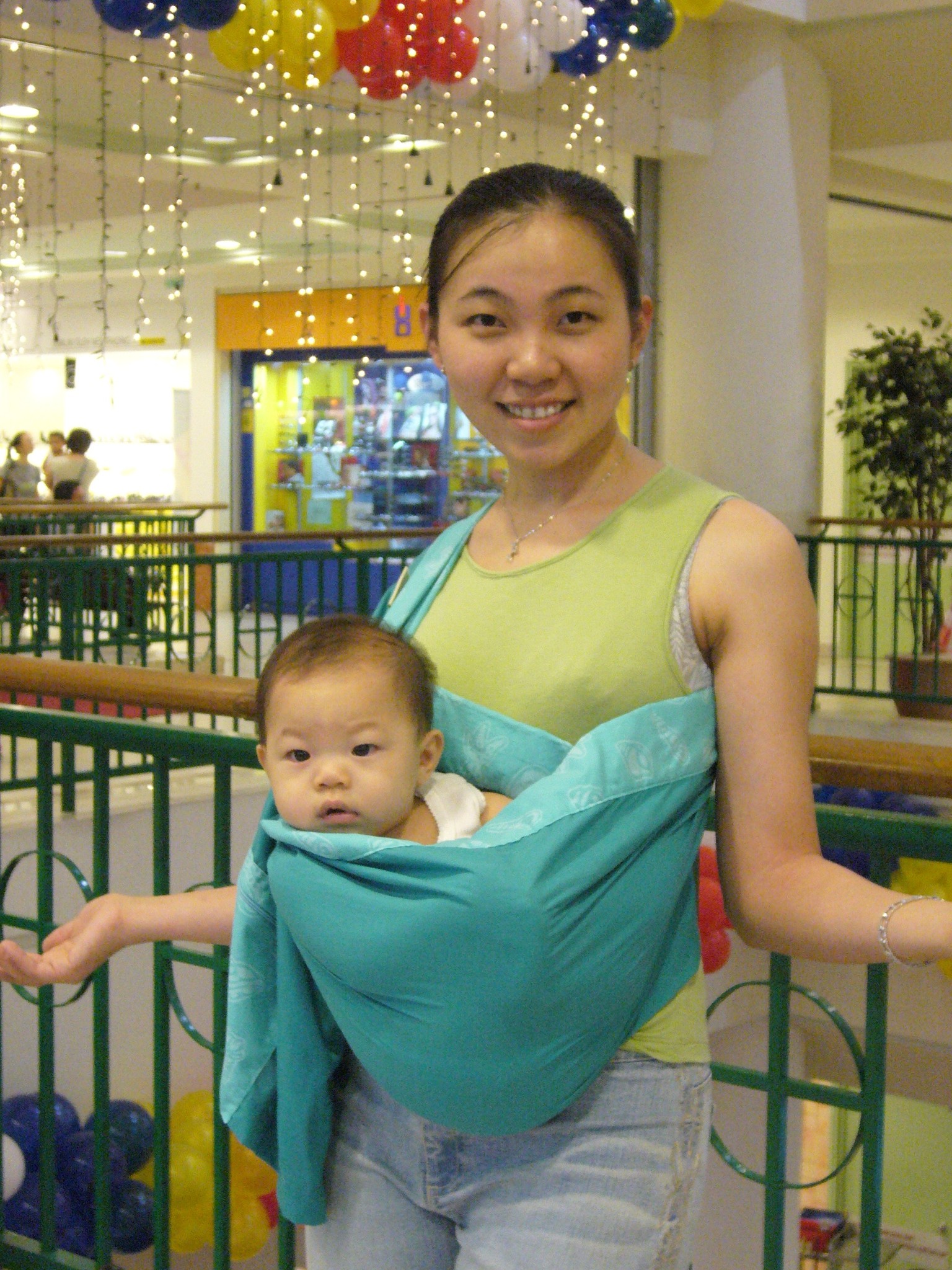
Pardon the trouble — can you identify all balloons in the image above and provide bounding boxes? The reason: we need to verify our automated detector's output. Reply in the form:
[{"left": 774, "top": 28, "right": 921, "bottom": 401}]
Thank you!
[{"left": 86, "top": 0, "right": 724, "bottom": 99}]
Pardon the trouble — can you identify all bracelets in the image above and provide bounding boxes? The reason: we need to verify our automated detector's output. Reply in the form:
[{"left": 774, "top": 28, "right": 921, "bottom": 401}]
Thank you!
[{"left": 875, "top": 892, "right": 940, "bottom": 977}]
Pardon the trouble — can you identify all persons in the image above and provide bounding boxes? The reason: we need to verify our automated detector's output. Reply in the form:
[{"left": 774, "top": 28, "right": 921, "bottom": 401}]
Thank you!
[
  {"left": 0, "top": 427, "right": 99, "bottom": 555},
  {"left": 0, "top": 155, "right": 952, "bottom": 1270},
  {"left": 252, "top": 615, "right": 522, "bottom": 857}
]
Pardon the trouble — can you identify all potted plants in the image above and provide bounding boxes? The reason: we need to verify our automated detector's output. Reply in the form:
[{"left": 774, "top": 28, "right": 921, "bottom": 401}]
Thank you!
[{"left": 828, "top": 306, "right": 952, "bottom": 720}]
